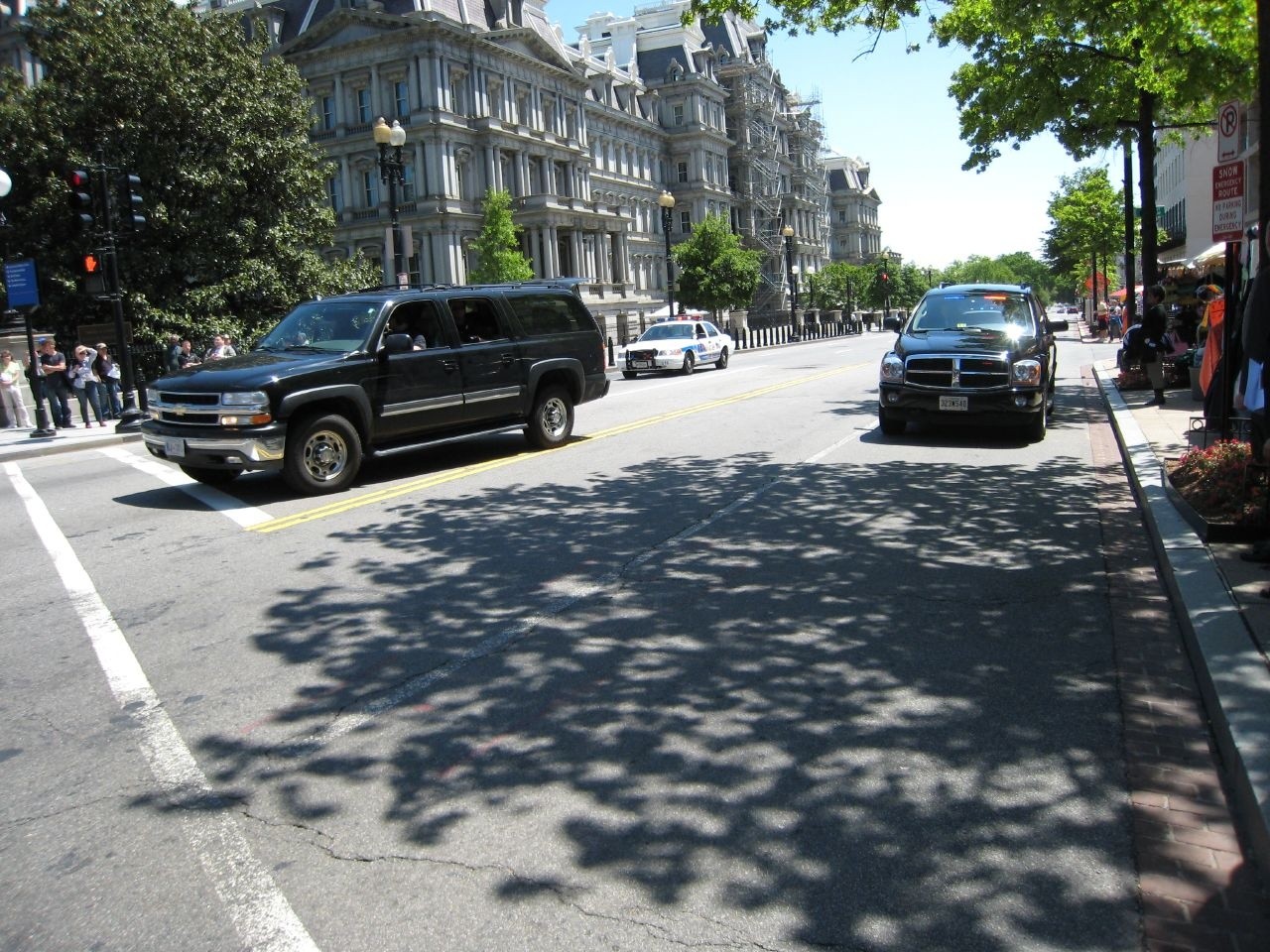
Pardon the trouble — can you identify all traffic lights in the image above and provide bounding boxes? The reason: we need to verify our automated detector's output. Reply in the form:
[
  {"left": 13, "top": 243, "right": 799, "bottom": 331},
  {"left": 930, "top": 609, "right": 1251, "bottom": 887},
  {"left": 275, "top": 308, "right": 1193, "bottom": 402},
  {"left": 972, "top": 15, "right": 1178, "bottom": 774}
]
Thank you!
[
  {"left": 81, "top": 252, "right": 107, "bottom": 292},
  {"left": 118, "top": 173, "right": 151, "bottom": 234},
  {"left": 68, "top": 169, "right": 99, "bottom": 234}
]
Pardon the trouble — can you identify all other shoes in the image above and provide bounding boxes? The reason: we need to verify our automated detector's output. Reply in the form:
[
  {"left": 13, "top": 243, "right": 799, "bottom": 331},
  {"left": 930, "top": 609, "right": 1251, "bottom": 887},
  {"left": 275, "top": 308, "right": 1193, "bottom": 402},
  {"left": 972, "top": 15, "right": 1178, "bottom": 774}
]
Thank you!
[
  {"left": 1145, "top": 399, "right": 1165, "bottom": 405},
  {"left": 102, "top": 413, "right": 111, "bottom": 421},
  {"left": 114, "top": 414, "right": 123, "bottom": 419},
  {"left": 86, "top": 422, "right": 91, "bottom": 428},
  {"left": 100, "top": 420, "right": 106, "bottom": 426},
  {"left": 56, "top": 423, "right": 63, "bottom": 429},
  {"left": 63, "top": 423, "right": 76, "bottom": 427}
]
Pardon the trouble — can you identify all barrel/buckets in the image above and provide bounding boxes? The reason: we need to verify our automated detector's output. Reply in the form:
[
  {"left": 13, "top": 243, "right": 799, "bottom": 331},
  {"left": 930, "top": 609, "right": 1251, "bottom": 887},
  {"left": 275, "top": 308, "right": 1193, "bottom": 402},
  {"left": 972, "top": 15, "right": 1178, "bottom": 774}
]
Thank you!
[{"left": 1188, "top": 365, "right": 1203, "bottom": 401}]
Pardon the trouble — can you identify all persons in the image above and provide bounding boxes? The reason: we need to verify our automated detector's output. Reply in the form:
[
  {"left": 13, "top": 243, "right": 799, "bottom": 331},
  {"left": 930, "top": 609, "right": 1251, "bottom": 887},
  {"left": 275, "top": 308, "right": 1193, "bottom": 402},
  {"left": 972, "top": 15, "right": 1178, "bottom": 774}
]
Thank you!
[
  {"left": 177, "top": 340, "right": 202, "bottom": 369},
  {"left": 1180, "top": 302, "right": 1205, "bottom": 344},
  {"left": 163, "top": 335, "right": 182, "bottom": 374},
  {"left": 67, "top": 345, "right": 107, "bottom": 429},
  {"left": 0, "top": 350, "right": 34, "bottom": 429},
  {"left": 1106, "top": 300, "right": 1128, "bottom": 343},
  {"left": 23, "top": 338, "right": 78, "bottom": 430},
  {"left": 93, "top": 343, "right": 123, "bottom": 421},
  {"left": 204, "top": 335, "right": 238, "bottom": 363},
  {"left": 1141, "top": 285, "right": 1169, "bottom": 404}
]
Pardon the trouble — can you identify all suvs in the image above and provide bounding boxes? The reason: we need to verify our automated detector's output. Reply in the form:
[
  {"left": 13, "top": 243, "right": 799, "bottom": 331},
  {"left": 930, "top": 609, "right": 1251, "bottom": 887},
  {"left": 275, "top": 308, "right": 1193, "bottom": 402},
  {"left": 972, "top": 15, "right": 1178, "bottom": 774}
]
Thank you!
[
  {"left": 143, "top": 279, "right": 615, "bottom": 497},
  {"left": 879, "top": 281, "right": 1069, "bottom": 445}
]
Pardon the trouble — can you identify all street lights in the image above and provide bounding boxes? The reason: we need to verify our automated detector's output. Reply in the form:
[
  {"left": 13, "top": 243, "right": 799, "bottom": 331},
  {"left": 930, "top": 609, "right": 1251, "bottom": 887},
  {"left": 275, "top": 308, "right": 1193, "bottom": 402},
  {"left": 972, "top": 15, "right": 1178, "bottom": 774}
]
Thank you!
[
  {"left": 782, "top": 223, "right": 802, "bottom": 342},
  {"left": 658, "top": 190, "right": 676, "bottom": 318},
  {"left": 880, "top": 250, "right": 896, "bottom": 320},
  {"left": 372, "top": 116, "right": 409, "bottom": 287}
]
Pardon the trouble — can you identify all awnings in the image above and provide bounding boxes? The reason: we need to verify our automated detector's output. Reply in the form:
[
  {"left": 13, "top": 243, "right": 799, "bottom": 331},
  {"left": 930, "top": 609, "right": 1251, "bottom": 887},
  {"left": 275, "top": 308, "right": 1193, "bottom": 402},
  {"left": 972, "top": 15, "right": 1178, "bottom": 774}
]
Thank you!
[
  {"left": 1110, "top": 286, "right": 1143, "bottom": 304},
  {"left": 1157, "top": 240, "right": 1225, "bottom": 280}
]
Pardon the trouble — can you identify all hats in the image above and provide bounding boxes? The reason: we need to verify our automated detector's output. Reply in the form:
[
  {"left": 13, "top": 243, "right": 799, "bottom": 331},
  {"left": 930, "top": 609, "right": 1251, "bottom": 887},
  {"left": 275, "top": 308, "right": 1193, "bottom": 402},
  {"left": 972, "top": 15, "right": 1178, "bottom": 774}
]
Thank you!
[
  {"left": 94, "top": 342, "right": 109, "bottom": 349},
  {"left": 38, "top": 336, "right": 48, "bottom": 345},
  {"left": 75, "top": 345, "right": 89, "bottom": 357},
  {"left": 1147, "top": 285, "right": 1167, "bottom": 296}
]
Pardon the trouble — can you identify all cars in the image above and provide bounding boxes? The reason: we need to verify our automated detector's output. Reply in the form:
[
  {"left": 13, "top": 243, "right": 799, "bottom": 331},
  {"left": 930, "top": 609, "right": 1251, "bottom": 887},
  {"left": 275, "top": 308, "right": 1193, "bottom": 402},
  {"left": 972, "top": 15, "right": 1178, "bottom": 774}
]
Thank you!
[
  {"left": 616, "top": 314, "right": 737, "bottom": 379},
  {"left": 1055, "top": 305, "right": 1079, "bottom": 315}
]
